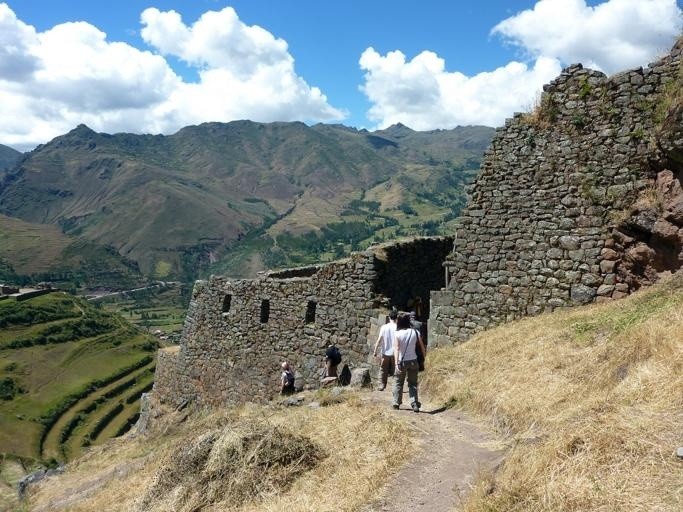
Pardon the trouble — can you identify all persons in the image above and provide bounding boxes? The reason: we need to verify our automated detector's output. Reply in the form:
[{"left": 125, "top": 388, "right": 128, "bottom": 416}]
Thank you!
[
  {"left": 279, "top": 362, "right": 294, "bottom": 397},
  {"left": 409, "top": 311, "right": 425, "bottom": 371},
  {"left": 324, "top": 340, "right": 337, "bottom": 377},
  {"left": 374, "top": 312, "right": 398, "bottom": 390},
  {"left": 392, "top": 313, "right": 425, "bottom": 412},
  {"left": 383, "top": 293, "right": 430, "bottom": 320}
]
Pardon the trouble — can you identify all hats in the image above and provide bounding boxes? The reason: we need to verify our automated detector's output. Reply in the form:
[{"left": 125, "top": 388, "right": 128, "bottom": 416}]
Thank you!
[{"left": 282, "top": 362, "right": 289, "bottom": 370}]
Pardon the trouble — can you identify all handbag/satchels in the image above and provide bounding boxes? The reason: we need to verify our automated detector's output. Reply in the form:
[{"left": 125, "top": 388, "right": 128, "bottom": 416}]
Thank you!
[{"left": 416, "top": 339, "right": 425, "bottom": 363}]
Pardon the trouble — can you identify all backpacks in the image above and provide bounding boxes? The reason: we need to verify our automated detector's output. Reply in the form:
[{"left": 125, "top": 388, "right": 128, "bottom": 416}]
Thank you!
[
  {"left": 335, "top": 351, "right": 342, "bottom": 364},
  {"left": 285, "top": 372, "right": 294, "bottom": 387}
]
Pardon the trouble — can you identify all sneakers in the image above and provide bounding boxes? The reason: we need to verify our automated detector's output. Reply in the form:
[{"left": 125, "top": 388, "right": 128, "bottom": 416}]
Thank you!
[
  {"left": 412, "top": 405, "right": 419, "bottom": 412},
  {"left": 393, "top": 405, "right": 399, "bottom": 409},
  {"left": 378, "top": 388, "right": 383, "bottom": 391}
]
